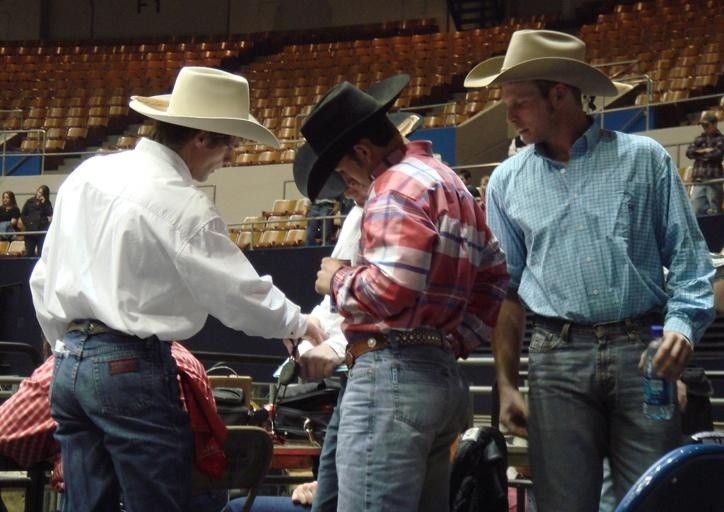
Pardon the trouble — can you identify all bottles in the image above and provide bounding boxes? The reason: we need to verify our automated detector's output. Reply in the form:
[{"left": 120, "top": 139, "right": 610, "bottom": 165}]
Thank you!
[{"left": 642, "top": 325, "right": 675, "bottom": 420}]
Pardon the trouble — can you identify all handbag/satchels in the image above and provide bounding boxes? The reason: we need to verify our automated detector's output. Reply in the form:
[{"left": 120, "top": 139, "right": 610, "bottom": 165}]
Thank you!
[
  {"left": 274, "top": 377, "right": 343, "bottom": 441},
  {"left": 210, "top": 388, "right": 269, "bottom": 427}
]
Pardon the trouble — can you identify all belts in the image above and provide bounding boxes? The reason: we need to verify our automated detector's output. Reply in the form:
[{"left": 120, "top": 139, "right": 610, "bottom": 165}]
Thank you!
[
  {"left": 65, "top": 319, "right": 120, "bottom": 336},
  {"left": 344, "top": 329, "right": 442, "bottom": 369}
]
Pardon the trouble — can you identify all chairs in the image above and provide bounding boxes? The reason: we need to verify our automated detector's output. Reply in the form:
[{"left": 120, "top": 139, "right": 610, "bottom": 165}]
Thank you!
[
  {"left": 0, "top": 0, "right": 724, "bottom": 256},
  {"left": 194, "top": 425, "right": 724, "bottom": 512}
]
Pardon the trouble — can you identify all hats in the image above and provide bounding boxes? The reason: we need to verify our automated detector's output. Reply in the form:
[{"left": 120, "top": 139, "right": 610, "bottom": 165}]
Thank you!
[
  {"left": 464, "top": 29, "right": 618, "bottom": 99},
  {"left": 293, "top": 75, "right": 424, "bottom": 204},
  {"left": 128, "top": 65, "right": 281, "bottom": 150},
  {"left": 696, "top": 109, "right": 718, "bottom": 126}
]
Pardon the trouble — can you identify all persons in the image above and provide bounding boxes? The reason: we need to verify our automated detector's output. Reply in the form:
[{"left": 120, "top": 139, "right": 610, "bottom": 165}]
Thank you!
[{"left": 2, "top": 28, "right": 723, "bottom": 512}]
